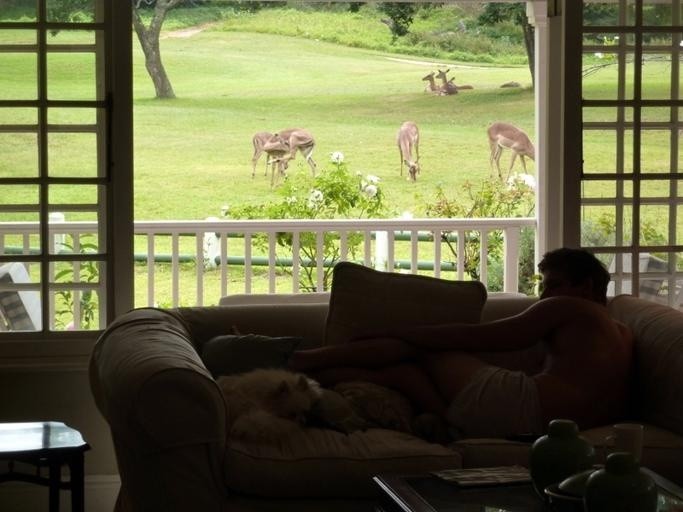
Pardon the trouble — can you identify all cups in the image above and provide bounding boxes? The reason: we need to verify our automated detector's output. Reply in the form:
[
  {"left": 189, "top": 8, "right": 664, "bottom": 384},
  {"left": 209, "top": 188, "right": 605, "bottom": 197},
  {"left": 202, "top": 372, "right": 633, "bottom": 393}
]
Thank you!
[{"left": 605, "top": 424, "right": 642, "bottom": 464}]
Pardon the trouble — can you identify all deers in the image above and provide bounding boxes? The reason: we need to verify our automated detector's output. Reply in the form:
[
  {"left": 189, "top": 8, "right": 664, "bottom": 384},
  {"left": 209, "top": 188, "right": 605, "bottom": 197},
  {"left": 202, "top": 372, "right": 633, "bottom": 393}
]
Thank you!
[
  {"left": 250, "top": 128, "right": 316, "bottom": 187},
  {"left": 398, "top": 121, "right": 420, "bottom": 181},
  {"left": 422, "top": 69, "right": 473, "bottom": 95},
  {"left": 487, "top": 121, "right": 535, "bottom": 181}
]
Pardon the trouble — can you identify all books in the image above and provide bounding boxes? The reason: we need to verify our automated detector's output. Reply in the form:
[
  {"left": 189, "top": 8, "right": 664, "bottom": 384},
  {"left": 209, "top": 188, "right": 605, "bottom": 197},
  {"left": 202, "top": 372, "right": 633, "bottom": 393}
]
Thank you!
[
  {"left": 429, "top": 464, "right": 532, "bottom": 489},
  {"left": 639, "top": 462, "right": 683, "bottom": 501}
]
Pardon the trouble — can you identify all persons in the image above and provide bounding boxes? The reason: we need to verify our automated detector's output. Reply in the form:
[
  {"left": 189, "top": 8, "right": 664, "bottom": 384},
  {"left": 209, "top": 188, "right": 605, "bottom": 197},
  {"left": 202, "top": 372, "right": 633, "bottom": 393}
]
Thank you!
[{"left": 231, "top": 248, "right": 637, "bottom": 449}]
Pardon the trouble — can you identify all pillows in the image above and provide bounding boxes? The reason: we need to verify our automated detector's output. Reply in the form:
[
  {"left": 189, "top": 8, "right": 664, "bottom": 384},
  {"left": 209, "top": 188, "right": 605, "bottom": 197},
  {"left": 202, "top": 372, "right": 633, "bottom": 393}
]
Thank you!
[
  {"left": 307, "top": 387, "right": 363, "bottom": 434},
  {"left": 201, "top": 332, "right": 308, "bottom": 380},
  {"left": 336, "top": 378, "right": 415, "bottom": 433},
  {"left": 321, "top": 262, "right": 487, "bottom": 347}
]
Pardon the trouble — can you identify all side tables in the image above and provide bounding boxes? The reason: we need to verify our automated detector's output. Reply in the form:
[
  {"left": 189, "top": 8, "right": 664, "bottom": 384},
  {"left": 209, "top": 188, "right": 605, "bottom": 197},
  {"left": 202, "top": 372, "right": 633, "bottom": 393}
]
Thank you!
[{"left": 0, "top": 420, "right": 91, "bottom": 512}]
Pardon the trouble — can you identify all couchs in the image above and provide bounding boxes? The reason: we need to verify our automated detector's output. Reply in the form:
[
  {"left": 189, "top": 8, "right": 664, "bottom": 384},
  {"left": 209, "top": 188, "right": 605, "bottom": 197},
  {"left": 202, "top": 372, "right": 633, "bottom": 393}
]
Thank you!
[{"left": 89, "top": 294, "right": 683, "bottom": 512}]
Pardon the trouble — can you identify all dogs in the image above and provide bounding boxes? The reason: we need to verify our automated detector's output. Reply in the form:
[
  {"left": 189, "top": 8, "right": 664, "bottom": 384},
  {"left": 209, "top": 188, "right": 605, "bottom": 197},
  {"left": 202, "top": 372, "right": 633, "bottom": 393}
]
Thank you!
[{"left": 216, "top": 368, "right": 324, "bottom": 440}]
